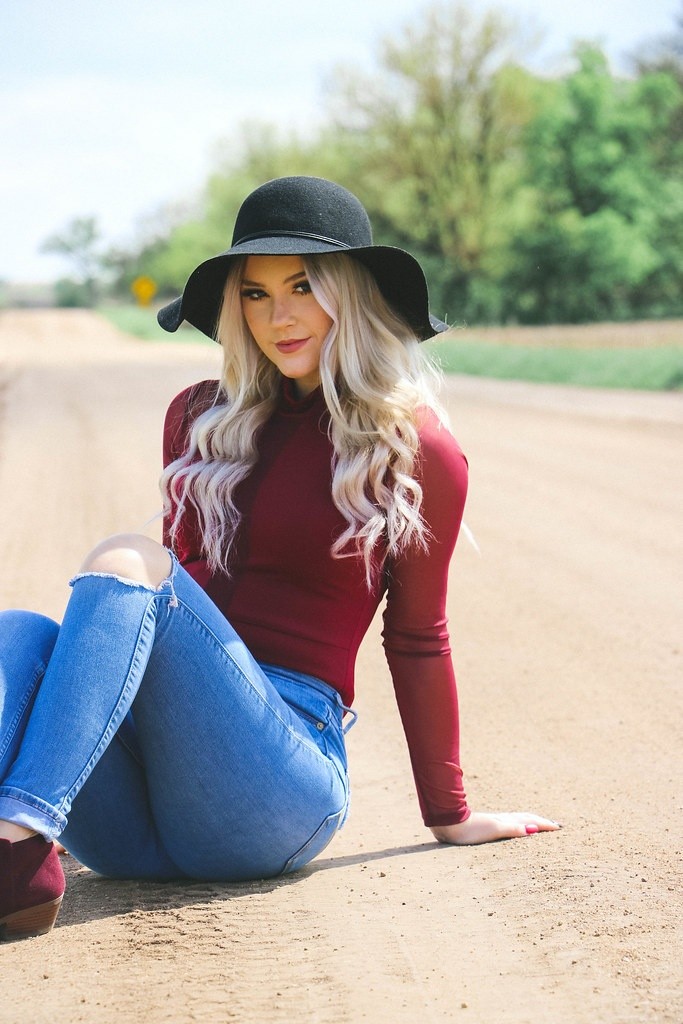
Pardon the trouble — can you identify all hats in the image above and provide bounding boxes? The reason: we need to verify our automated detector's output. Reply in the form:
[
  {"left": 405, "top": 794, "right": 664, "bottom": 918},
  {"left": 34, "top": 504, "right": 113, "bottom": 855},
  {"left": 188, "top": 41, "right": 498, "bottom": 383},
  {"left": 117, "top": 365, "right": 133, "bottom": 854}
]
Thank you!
[{"left": 157, "top": 177, "right": 449, "bottom": 346}]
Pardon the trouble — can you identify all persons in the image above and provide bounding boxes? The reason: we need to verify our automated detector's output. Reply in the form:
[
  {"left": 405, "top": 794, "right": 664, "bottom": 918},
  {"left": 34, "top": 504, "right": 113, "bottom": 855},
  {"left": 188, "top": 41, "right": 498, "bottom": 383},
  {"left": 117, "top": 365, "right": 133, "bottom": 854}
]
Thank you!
[{"left": 0, "top": 175, "right": 563, "bottom": 942}]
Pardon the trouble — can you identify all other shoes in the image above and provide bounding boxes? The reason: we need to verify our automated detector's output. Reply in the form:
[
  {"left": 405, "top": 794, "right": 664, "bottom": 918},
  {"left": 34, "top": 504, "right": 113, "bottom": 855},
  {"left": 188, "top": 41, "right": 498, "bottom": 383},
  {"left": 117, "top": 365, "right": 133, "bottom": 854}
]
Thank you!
[{"left": 1, "top": 834, "right": 66, "bottom": 938}]
